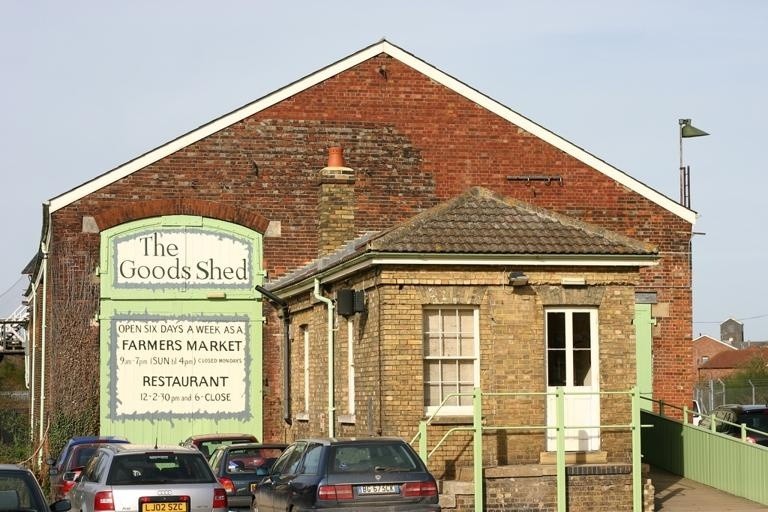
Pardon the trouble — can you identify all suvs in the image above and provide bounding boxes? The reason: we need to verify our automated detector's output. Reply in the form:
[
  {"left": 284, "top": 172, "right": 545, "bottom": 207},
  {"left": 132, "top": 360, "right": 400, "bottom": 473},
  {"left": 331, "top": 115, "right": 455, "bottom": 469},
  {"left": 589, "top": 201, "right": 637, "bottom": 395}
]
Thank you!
[{"left": 699, "top": 403, "right": 767, "bottom": 444}]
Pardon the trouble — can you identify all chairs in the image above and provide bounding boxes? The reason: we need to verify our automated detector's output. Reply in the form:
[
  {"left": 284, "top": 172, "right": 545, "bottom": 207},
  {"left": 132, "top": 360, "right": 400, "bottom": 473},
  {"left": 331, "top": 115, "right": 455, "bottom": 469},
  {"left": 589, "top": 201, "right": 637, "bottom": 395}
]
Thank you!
[
  {"left": 117, "top": 466, "right": 189, "bottom": 481},
  {"left": 0, "top": 490, "right": 20, "bottom": 512},
  {"left": 229, "top": 457, "right": 278, "bottom": 472},
  {"left": 200, "top": 446, "right": 209, "bottom": 455}
]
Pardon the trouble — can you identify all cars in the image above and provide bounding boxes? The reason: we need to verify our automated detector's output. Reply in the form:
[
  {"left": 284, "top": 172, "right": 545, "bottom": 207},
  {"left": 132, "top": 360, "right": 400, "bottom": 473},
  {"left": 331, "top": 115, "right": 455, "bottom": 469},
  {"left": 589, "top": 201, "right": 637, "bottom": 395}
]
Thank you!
[{"left": 692, "top": 399, "right": 701, "bottom": 426}]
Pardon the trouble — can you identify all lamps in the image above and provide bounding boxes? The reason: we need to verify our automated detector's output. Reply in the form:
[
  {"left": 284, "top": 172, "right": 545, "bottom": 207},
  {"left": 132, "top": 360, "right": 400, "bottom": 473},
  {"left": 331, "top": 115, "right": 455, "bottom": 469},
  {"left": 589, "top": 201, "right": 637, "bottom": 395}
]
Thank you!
[{"left": 508, "top": 272, "right": 529, "bottom": 287}]
